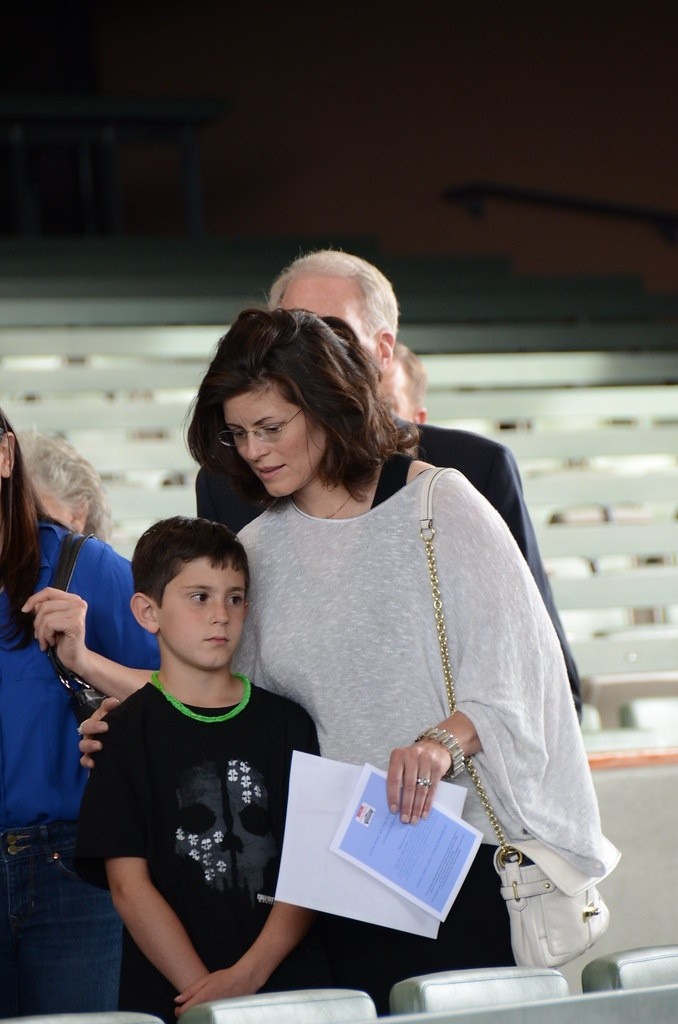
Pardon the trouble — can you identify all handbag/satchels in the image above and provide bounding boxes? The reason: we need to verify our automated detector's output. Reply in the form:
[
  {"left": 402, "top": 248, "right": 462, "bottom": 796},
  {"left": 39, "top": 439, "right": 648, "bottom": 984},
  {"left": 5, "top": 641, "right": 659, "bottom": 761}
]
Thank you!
[{"left": 494, "top": 840, "right": 608, "bottom": 970}]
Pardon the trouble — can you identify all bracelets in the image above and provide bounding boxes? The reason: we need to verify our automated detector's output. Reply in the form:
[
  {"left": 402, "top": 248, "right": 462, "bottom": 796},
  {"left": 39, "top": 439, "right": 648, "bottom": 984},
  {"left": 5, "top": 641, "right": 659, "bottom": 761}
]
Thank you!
[{"left": 416, "top": 727, "right": 465, "bottom": 782}]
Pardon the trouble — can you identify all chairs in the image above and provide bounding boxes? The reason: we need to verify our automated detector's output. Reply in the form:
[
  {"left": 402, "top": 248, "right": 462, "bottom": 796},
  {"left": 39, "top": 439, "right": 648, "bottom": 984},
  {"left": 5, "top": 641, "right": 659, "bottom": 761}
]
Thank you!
[{"left": 0, "top": 453, "right": 678, "bottom": 1024}]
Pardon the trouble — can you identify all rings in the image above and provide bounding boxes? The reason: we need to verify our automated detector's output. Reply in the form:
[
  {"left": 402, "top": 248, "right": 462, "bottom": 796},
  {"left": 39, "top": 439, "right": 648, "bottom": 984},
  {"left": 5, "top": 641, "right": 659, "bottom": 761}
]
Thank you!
[
  {"left": 77, "top": 719, "right": 88, "bottom": 735},
  {"left": 417, "top": 778, "right": 431, "bottom": 786}
]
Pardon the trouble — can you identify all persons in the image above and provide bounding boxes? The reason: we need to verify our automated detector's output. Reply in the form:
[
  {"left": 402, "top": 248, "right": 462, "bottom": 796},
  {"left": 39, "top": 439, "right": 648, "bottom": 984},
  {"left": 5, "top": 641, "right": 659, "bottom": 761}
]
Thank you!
[
  {"left": 373, "top": 343, "right": 429, "bottom": 422},
  {"left": 13, "top": 427, "right": 114, "bottom": 543},
  {"left": 0, "top": 409, "right": 166, "bottom": 1019},
  {"left": 195, "top": 248, "right": 584, "bottom": 728},
  {"left": 69, "top": 516, "right": 322, "bottom": 1024},
  {"left": 78, "top": 307, "right": 623, "bottom": 1009}
]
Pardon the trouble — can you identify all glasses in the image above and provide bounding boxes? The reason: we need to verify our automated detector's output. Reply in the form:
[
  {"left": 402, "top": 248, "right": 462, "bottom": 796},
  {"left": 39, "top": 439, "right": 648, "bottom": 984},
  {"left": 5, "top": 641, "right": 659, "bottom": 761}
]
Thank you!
[{"left": 217, "top": 407, "right": 304, "bottom": 446}]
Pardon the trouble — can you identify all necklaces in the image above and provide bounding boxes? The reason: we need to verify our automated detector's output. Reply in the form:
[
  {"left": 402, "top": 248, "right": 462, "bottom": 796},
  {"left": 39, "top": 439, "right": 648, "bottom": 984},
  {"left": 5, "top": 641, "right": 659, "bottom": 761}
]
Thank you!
[
  {"left": 152, "top": 670, "right": 250, "bottom": 723},
  {"left": 325, "top": 495, "right": 353, "bottom": 520}
]
